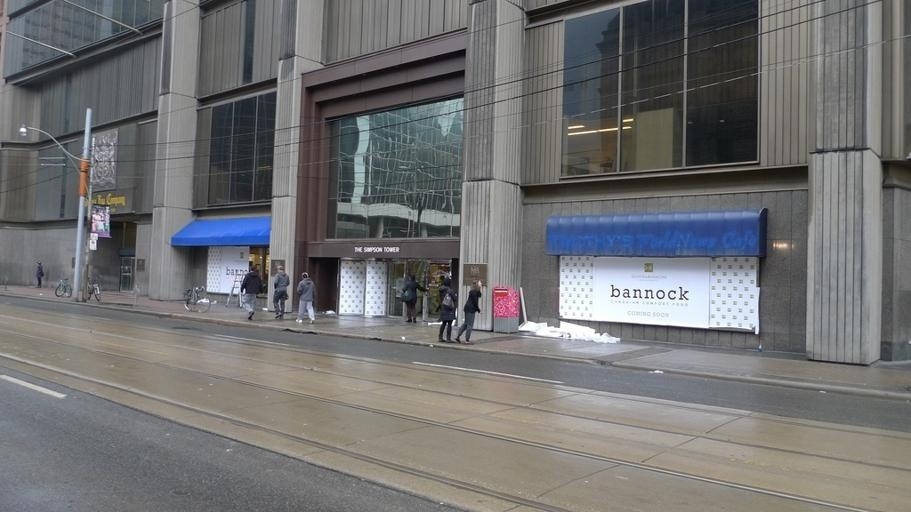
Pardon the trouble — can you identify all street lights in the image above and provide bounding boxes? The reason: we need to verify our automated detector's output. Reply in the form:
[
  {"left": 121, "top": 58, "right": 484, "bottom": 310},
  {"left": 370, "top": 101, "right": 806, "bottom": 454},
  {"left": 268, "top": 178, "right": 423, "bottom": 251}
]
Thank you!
[{"left": 20, "top": 106, "right": 95, "bottom": 303}]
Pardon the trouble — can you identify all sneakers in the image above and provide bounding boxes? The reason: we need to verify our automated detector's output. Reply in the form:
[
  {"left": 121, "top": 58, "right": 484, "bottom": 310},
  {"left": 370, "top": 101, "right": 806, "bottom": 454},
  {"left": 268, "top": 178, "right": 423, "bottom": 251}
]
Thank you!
[
  {"left": 275, "top": 311, "right": 282, "bottom": 318},
  {"left": 405, "top": 318, "right": 417, "bottom": 323},
  {"left": 439, "top": 339, "right": 471, "bottom": 345},
  {"left": 248, "top": 311, "right": 254, "bottom": 319},
  {"left": 295, "top": 319, "right": 302, "bottom": 323}
]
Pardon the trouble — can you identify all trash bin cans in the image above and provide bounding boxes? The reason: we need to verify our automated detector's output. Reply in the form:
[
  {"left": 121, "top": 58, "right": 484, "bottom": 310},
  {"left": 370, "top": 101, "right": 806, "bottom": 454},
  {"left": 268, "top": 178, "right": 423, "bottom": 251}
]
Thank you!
[{"left": 493, "top": 286, "right": 520, "bottom": 334}]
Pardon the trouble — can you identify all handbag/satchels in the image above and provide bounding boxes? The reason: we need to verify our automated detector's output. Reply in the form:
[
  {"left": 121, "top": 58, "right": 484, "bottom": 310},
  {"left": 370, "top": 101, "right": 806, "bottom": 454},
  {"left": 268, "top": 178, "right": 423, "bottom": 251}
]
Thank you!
[
  {"left": 441, "top": 293, "right": 455, "bottom": 310},
  {"left": 401, "top": 288, "right": 413, "bottom": 302}
]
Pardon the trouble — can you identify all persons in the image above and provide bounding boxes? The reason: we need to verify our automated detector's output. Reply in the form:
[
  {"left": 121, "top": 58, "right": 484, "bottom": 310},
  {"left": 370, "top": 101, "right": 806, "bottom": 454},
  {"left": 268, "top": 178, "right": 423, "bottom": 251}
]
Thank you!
[
  {"left": 296, "top": 272, "right": 317, "bottom": 324},
  {"left": 35, "top": 262, "right": 44, "bottom": 288},
  {"left": 438, "top": 277, "right": 458, "bottom": 343},
  {"left": 273, "top": 266, "right": 290, "bottom": 319},
  {"left": 402, "top": 274, "right": 429, "bottom": 323},
  {"left": 455, "top": 280, "right": 482, "bottom": 344},
  {"left": 241, "top": 265, "right": 264, "bottom": 320}
]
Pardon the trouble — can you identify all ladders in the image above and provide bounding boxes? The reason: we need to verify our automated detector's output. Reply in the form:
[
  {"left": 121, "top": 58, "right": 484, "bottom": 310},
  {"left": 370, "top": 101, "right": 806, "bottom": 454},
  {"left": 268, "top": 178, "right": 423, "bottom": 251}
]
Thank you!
[{"left": 225, "top": 269, "right": 244, "bottom": 308}]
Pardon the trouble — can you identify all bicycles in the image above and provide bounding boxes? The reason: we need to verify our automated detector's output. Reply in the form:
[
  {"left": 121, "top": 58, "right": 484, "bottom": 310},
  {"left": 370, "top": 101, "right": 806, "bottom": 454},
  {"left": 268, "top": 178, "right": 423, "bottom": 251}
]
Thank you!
[
  {"left": 183, "top": 287, "right": 213, "bottom": 313},
  {"left": 88, "top": 283, "right": 101, "bottom": 303},
  {"left": 55, "top": 279, "right": 72, "bottom": 297}
]
